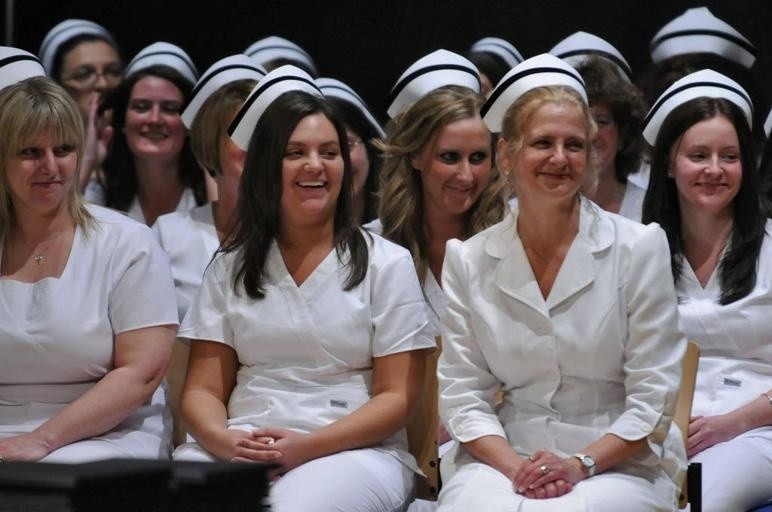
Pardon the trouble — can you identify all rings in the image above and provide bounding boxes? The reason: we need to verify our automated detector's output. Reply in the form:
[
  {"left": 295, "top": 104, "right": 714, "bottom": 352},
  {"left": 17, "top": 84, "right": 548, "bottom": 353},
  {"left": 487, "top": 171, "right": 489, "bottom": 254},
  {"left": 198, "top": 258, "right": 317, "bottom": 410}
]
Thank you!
[
  {"left": 527, "top": 455, "right": 535, "bottom": 462},
  {"left": 540, "top": 465, "right": 549, "bottom": 473}
]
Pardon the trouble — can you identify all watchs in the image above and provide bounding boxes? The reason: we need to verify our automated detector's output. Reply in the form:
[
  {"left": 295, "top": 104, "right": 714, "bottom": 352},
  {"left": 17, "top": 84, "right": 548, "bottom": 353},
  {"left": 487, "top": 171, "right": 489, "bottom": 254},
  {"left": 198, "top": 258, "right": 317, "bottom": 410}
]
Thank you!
[{"left": 573, "top": 451, "right": 596, "bottom": 476}]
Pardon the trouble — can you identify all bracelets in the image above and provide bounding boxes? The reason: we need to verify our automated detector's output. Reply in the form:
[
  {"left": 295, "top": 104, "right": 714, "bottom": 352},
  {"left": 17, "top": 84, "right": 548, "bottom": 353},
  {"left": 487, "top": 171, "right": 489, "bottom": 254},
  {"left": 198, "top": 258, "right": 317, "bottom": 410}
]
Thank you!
[{"left": 758, "top": 391, "right": 771, "bottom": 404}]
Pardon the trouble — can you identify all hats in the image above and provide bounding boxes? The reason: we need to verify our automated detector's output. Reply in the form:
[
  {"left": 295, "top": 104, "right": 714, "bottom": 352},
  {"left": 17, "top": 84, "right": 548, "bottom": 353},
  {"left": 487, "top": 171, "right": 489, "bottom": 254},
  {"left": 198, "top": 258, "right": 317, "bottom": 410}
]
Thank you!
[
  {"left": 242, "top": 35, "right": 318, "bottom": 77},
  {"left": 314, "top": 77, "right": 387, "bottom": 142},
  {"left": 548, "top": 31, "right": 637, "bottom": 87},
  {"left": 38, "top": 18, "right": 119, "bottom": 77},
  {"left": 226, "top": 63, "right": 329, "bottom": 153},
  {"left": 471, "top": 36, "right": 525, "bottom": 69},
  {"left": 648, "top": 6, "right": 759, "bottom": 70},
  {"left": 122, "top": 40, "right": 200, "bottom": 86},
  {"left": 480, "top": 51, "right": 590, "bottom": 134},
  {"left": 0, "top": 43, "right": 48, "bottom": 93},
  {"left": 639, "top": 68, "right": 754, "bottom": 147},
  {"left": 386, "top": 48, "right": 481, "bottom": 125},
  {"left": 178, "top": 54, "right": 268, "bottom": 132}
]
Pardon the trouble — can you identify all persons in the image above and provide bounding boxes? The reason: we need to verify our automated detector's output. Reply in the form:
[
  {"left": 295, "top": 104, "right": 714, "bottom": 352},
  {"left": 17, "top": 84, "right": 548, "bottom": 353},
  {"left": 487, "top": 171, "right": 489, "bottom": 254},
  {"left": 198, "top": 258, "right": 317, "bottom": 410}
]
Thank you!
[
  {"left": 354, "top": 82, "right": 512, "bottom": 501},
  {"left": 35, "top": 5, "right": 772, "bottom": 234},
  {"left": 632, "top": 67, "right": 771, "bottom": 511},
  {"left": 0, "top": 48, "right": 179, "bottom": 463},
  {"left": 420, "top": 51, "right": 694, "bottom": 510},
  {"left": 134, "top": 51, "right": 275, "bottom": 458},
  {"left": 166, "top": 90, "right": 443, "bottom": 511}
]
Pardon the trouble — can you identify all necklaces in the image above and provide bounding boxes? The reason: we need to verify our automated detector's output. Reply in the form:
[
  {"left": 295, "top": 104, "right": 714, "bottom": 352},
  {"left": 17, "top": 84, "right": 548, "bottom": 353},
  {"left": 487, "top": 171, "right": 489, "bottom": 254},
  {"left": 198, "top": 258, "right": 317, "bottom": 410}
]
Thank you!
[{"left": 10, "top": 217, "right": 74, "bottom": 269}]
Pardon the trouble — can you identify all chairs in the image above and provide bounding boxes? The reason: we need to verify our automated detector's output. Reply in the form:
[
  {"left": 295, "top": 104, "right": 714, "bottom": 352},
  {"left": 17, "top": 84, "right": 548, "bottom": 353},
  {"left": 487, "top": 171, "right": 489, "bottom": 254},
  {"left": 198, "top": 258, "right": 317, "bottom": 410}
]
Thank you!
[
  {"left": 411, "top": 333, "right": 445, "bottom": 506},
  {"left": 672, "top": 339, "right": 702, "bottom": 511},
  {"left": 160, "top": 322, "right": 192, "bottom": 456}
]
